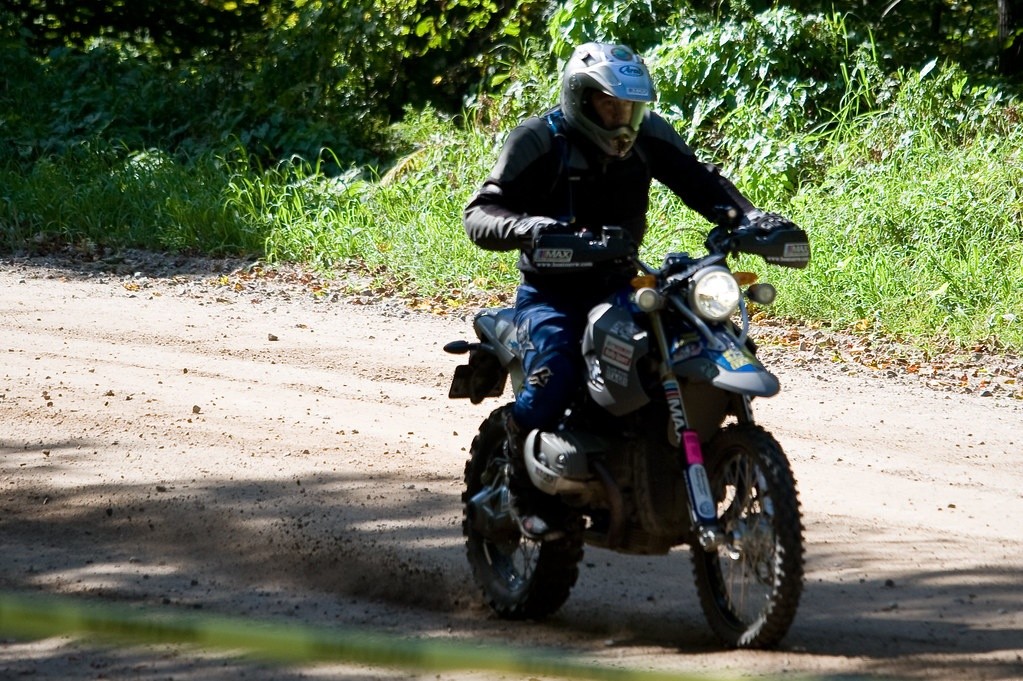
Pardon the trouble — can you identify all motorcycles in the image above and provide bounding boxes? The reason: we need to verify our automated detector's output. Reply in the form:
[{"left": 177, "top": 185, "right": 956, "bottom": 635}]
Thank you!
[{"left": 443, "top": 228, "right": 804, "bottom": 648}]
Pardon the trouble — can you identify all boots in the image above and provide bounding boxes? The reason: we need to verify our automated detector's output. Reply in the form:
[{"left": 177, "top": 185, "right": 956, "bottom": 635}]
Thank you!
[{"left": 499, "top": 408, "right": 563, "bottom": 539}]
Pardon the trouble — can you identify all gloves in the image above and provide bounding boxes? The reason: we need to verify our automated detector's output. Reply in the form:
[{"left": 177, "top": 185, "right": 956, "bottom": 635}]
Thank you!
[
  {"left": 738, "top": 206, "right": 810, "bottom": 268},
  {"left": 527, "top": 218, "right": 574, "bottom": 257}
]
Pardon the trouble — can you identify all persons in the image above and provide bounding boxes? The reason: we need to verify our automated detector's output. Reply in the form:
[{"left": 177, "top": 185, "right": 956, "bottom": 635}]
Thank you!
[{"left": 463, "top": 42, "right": 810, "bottom": 540}]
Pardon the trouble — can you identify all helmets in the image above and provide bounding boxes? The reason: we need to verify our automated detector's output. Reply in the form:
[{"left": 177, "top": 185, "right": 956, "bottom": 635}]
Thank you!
[{"left": 559, "top": 41, "right": 654, "bottom": 157}]
